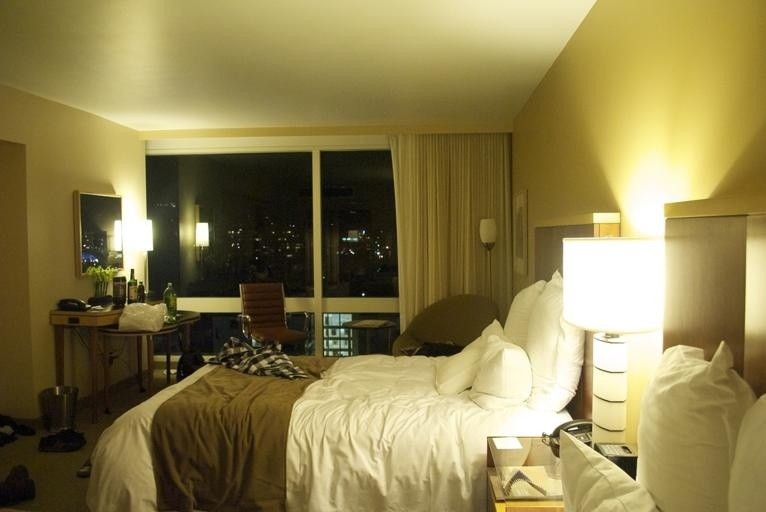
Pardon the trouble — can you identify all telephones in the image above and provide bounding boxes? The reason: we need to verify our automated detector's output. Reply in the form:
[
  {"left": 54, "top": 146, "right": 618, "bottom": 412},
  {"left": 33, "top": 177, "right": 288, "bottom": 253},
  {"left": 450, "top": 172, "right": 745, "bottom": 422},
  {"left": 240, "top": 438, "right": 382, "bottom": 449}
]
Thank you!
[
  {"left": 58, "top": 299, "right": 91, "bottom": 311},
  {"left": 549, "top": 418, "right": 592, "bottom": 447}
]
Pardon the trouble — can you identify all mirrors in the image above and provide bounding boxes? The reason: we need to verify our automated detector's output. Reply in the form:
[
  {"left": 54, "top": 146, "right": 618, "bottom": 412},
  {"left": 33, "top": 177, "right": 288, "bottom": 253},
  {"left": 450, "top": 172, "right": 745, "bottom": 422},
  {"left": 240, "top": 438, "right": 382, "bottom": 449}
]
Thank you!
[{"left": 73, "top": 191, "right": 124, "bottom": 278}]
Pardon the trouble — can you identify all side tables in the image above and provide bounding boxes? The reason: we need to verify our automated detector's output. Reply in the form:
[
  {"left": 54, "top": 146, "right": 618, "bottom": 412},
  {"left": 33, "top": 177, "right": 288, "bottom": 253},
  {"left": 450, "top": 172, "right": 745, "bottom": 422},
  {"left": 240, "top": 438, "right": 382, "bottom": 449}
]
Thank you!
[{"left": 343, "top": 320, "right": 396, "bottom": 356}]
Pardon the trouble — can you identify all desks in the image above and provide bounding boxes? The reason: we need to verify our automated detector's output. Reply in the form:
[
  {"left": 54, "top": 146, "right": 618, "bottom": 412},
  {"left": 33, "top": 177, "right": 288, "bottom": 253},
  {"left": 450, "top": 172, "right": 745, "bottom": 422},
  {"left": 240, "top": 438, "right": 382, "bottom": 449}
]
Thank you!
[{"left": 49, "top": 302, "right": 201, "bottom": 427}]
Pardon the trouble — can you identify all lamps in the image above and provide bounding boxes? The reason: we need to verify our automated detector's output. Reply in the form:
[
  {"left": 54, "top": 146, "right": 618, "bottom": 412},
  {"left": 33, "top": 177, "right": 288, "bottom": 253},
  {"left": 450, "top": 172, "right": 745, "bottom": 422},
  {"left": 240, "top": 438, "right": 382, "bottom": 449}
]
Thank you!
[
  {"left": 480, "top": 219, "right": 497, "bottom": 301},
  {"left": 195, "top": 222, "right": 209, "bottom": 280},
  {"left": 563, "top": 238, "right": 667, "bottom": 449},
  {"left": 112, "top": 220, "right": 123, "bottom": 253},
  {"left": 123, "top": 220, "right": 153, "bottom": 292}
]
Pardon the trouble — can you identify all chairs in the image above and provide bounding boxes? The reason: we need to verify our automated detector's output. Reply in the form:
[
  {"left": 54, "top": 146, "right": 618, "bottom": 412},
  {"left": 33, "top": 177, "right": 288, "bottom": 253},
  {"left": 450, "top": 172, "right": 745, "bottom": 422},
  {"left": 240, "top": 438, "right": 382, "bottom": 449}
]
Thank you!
[
  {"left": 238, "top": 282, "right": 310, "bottom": 353},
  {"left": 394, "top": 294, "right": 499, "bottom": 356}
]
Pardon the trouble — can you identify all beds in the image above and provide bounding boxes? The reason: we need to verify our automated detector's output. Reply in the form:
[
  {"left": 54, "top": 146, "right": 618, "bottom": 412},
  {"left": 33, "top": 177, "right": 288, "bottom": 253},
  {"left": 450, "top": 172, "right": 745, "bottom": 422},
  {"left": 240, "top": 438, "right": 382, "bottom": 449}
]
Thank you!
[
  {"left": 86, "top": 213, "right": 620, "bottom": 512},
  {"left": 662, "top": 193, "right": 766, "bottom": 380}
]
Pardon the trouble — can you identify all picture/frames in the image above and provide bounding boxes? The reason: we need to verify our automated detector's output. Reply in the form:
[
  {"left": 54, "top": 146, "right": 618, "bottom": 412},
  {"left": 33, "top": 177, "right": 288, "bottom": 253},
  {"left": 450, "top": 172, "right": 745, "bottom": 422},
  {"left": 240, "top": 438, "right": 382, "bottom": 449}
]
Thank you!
[{"left": 512, "top": 189, "right": 527, "bottom": 275}]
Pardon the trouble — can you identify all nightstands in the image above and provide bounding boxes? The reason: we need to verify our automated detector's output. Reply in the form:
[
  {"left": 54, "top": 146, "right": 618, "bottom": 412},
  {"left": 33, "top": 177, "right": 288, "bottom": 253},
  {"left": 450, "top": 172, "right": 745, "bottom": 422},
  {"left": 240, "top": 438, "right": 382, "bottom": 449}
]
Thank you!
[{"left": 487, "top": 438, "right": 564, "bottom": 512}]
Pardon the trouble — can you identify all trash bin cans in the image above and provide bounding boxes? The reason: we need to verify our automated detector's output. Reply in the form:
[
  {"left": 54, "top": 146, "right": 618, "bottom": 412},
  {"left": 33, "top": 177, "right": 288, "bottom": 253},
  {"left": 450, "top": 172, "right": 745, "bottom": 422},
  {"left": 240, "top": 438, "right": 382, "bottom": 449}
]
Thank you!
[{"left": 37, "top": 384, "right": 79, "bottom": 433}]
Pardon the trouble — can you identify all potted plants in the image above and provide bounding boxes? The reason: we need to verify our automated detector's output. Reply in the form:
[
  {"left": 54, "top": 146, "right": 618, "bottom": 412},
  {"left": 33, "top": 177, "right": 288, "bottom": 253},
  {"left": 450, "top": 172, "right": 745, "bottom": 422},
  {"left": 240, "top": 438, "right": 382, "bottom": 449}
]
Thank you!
[{"left": 87, "top": 266, "right": 120, "bottom": 306}]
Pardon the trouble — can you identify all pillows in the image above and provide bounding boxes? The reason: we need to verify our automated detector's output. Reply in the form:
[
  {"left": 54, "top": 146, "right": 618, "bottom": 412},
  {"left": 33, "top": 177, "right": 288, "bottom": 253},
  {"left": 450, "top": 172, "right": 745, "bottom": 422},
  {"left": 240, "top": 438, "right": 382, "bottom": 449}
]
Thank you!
[
  {"left": 728, "top": 393, "right": 766, "bottom": 512},
  {"left": 559, "top": 430, "right": 660, "bottom": 512},
  {"left": 468, "top": 335, "right": 532, "bottom": 409},
  {"left": 504, "top": 280, "right": 546, "bottom": 347},
  {"left": 636, "top": 341, "right": 756, "bottom": 512},
  {"left": 436, "top": 319, "right": 513, "bottom": 395}
]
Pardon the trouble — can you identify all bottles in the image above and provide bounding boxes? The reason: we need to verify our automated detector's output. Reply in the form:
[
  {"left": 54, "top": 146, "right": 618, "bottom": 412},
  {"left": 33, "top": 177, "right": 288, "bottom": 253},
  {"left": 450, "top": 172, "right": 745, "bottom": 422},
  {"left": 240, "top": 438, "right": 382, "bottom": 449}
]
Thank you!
[
  {"left": 137, "top": 282, "right": 145, "bottom": 302},
  {"left": 162, "top": 282, "right": 178, "bottom": 324},
  {"left": 127, "top": 268, "right": 138, "bottom": 304},
  {"left": 112, "top": 276, "right": 126, "bottom": 305}
]
